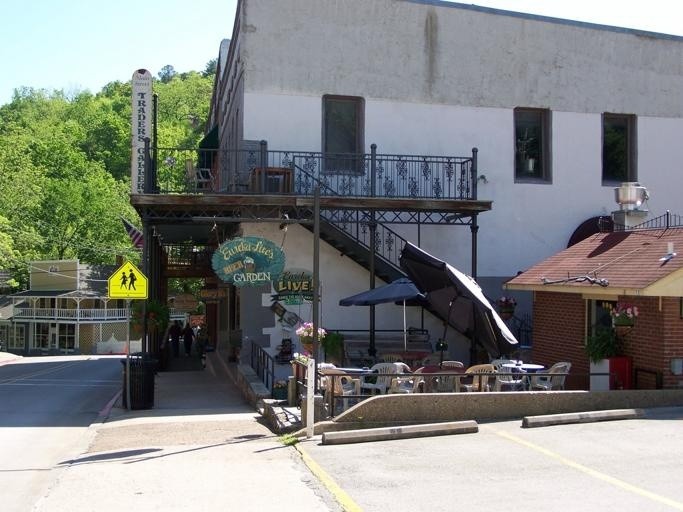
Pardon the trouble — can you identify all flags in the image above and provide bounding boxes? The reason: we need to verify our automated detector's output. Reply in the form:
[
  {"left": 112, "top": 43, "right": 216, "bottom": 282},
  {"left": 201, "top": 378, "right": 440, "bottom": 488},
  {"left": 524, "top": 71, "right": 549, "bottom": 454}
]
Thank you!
[{"left": 120, "top": 217, "right": 142, "bottom": 248}]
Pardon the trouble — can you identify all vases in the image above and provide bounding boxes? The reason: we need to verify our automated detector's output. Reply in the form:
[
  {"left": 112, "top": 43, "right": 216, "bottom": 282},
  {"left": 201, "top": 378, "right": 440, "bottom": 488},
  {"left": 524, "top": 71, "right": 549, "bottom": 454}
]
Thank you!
[
  {"left": 303, "top": 343, "right": 321, "bottom": 355},
  {"left": 273, "top": 389, "right": 287, "bottom": 399},
  {"left": 613, "top": 325, "right": 633, "bottom": 336}
]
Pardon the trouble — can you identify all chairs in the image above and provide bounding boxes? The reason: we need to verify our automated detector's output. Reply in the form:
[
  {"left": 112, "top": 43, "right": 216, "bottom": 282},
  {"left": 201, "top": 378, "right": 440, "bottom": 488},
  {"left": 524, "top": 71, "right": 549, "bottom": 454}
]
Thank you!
[
  {"left": 320, "top": 354, "right": 573, "bottom": 414},
  {"left": 185, "top": 158, "right": 216, "bottom": 193}
]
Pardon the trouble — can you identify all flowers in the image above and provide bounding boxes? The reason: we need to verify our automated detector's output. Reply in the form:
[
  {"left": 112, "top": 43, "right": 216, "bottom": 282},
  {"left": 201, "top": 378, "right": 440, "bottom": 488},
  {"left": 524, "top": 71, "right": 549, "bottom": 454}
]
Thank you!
[
  {"left": 296, "top": 322, "right": 327, "bottom": 342},
  {"left": 608, "top": 303, "right": 639, "bottom": 324},
  {"left": 273, "top": 380, "right": 286, "bottom": 389}
]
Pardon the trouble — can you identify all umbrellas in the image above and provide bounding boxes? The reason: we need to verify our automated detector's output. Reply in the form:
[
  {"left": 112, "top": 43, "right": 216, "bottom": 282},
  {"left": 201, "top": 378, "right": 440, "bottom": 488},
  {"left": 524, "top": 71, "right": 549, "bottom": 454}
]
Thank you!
[
  {"left": 398, "top": 240, "right": 518, "bottom": 369},
  {"left": 335, "top": 275, "right": 424, "bottom": 351}
]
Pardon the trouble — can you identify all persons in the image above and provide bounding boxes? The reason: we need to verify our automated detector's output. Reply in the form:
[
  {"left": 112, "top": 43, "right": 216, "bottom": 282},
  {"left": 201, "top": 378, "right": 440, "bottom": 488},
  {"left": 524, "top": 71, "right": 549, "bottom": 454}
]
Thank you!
[
  {"left": 167, "top": 318, "right": 181, "bottom": 358},
  {"left": 180, "top": 321, "right": 195, "bottom": 356}
]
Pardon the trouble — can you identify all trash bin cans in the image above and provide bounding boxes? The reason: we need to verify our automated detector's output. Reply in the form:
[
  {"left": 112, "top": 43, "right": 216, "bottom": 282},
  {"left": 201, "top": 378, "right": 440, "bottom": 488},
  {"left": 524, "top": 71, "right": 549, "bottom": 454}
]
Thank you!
[{"left": 120, "top": 359, "right": 159, "bottom": 410}]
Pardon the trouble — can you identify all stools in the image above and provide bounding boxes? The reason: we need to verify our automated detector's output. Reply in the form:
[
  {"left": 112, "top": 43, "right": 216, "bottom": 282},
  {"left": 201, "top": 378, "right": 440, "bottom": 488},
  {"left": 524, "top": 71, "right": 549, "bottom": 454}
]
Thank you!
[{"left": 254, "top": 167, "right": 292, "bottom": 194}]
